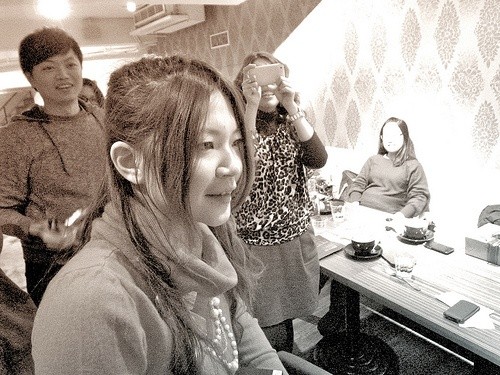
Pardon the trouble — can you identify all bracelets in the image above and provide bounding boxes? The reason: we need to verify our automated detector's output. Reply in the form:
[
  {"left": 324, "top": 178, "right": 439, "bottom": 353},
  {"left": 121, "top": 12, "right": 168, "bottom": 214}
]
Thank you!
[{"left": 289, "top": 110, "right": 307, "bottom": 122}]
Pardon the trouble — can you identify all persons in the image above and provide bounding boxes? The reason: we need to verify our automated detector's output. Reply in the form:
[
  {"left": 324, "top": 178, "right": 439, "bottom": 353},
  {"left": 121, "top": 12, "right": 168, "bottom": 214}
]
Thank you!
[
  {"left": 31, "top": 55, "right": 288, "bottom": 375},
  {"left": 0, "top": 28, "right": 111, "bottom": 310},
  {"left": 78, "top": 78, "right": 104, "bottom": 108},
  {"left": 233, "top": 51, "right": 328, "bottom": 375},
  {"left": 317, "top": 116, "right": 430, "bottom": 336}
]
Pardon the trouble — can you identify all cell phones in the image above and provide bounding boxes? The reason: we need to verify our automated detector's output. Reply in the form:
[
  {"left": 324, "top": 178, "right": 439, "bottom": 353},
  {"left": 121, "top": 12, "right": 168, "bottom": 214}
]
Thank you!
[
  {"left": 248, "top": 63, "right": 285, "bottom": 86},
  {"left": 423, "top": 240, "right": 454, "bottom": 254}
]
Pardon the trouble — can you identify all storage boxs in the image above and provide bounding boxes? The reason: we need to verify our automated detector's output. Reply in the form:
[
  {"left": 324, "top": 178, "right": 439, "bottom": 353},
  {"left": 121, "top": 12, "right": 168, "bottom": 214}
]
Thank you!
[{"left": 465, "top": 223, "right": 500, "bottom": 266}]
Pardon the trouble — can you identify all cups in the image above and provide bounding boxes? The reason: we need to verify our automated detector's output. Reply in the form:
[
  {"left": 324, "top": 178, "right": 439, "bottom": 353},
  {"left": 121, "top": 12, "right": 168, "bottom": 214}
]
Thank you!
[
  {"left": 395, "top": 254, "right": 416, "bottom": 278},
  {"left": 330, "top": 201, "right": 345, "bottom": 222},
  {"left": 351, "top": 240, "right": 374, "bottom": 256},
  {"left": 404, "top": 224, "right": 428, "bottom": 239}
]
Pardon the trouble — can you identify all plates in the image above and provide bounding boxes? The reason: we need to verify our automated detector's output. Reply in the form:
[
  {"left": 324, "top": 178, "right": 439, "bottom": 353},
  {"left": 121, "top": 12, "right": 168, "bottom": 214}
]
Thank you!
[
  {"left": 320, "top": 200, "right": 331, "bottom": 215},
  {"left": 344, "top": 244, "right": 382, "bottom": 259},
  {"left": 398, "top": 229, "right": 437, "bottom": 243}
]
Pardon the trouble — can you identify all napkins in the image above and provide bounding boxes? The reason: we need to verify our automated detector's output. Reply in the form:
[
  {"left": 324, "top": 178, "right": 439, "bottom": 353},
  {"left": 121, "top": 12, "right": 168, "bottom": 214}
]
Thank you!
[{"left": 437, "top": 290, "right": 496, "bottom": 329}]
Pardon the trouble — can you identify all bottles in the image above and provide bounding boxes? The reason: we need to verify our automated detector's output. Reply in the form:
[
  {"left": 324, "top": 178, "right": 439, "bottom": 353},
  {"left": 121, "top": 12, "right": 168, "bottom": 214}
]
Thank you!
[{"left": 308, "top": 177, "right": 320, "bottom": 220}]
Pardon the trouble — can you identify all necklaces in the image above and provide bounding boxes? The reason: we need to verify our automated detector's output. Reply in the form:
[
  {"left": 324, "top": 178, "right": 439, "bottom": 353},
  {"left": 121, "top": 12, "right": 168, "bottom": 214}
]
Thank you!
[{"left": 156, "top": 293, "right": 239, "bottom": 373}]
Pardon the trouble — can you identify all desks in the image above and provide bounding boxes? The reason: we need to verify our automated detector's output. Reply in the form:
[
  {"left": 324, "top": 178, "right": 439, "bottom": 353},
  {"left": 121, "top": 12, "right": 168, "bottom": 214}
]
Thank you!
[{"left": 308, "top": 195, "right": 500, "bottom": 375}]
